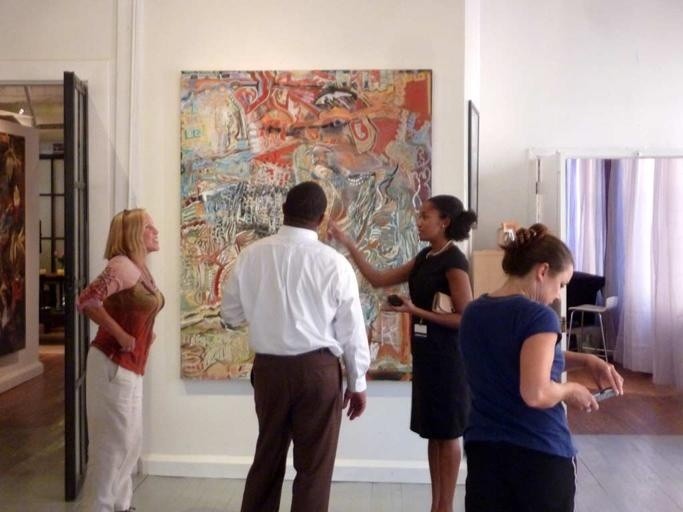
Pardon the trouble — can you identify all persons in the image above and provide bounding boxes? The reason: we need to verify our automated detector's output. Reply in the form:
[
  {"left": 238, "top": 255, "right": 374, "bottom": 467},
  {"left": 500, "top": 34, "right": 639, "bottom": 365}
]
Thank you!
[
  {"left": 68, "top": 208, "right": 165, "bottom": 512},
  {"left": 220, "top": 181, "right": 370, "bottom": 512},
  {"left": 459, "top": 222, "right": 623, "bottom": 511},
  {"left": 331, "top": 194, "right": 476, "bottom": 511}
]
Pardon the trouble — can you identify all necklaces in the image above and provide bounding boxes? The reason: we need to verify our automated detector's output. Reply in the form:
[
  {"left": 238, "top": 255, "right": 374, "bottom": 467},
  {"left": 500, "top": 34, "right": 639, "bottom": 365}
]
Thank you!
[{"left": 424, "top": 240, "right": 452, "bottom": 259}]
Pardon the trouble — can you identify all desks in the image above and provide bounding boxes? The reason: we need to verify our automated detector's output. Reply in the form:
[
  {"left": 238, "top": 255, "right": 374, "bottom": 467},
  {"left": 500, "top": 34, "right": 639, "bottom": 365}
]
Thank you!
[{"left": 39, "top": 274, "right": 66, "bottom": 333}]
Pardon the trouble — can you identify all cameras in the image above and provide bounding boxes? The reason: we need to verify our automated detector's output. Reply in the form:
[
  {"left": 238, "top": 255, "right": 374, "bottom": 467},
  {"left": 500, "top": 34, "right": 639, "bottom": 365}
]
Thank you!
[{"left": 591, "top": 387, "right": 618, "bottom": 403}]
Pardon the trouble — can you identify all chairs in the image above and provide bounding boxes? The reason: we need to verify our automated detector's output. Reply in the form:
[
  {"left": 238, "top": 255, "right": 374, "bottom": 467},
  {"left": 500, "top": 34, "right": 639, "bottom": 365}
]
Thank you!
[{"left": 566, "top": 296, "right": 619, "bottom": 363}]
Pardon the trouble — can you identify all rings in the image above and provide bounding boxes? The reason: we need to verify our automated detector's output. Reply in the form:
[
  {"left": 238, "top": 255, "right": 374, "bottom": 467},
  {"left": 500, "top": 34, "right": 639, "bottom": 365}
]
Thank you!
[{"left": 127, "top": 346, "right": 131, "bottom": 350}]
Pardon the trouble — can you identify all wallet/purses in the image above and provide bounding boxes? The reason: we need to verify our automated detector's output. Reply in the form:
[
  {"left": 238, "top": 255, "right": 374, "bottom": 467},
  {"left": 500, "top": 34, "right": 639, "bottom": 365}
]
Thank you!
[{"left": 432, "top": 291, "right": 455, "bottom": 314}]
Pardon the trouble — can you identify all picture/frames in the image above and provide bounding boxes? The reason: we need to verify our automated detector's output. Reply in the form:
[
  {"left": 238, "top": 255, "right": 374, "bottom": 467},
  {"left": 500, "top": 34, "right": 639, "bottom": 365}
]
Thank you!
[{"left": 467, "top": 100, "right": 480, "bottom": 223}]
